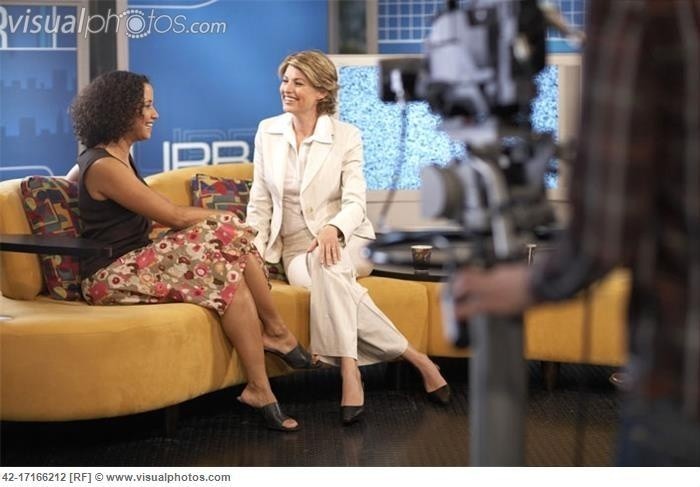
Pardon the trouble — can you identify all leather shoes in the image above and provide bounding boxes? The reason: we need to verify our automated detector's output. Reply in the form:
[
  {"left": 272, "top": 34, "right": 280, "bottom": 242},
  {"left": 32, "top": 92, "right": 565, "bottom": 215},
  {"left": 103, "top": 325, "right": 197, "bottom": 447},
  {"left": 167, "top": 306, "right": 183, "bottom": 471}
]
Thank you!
[
  {"left": 340, "top": 370, "right": 368, "bottom": 423},
  {"left": 427, "top": 364, "right": 451, "bottom": 406}
]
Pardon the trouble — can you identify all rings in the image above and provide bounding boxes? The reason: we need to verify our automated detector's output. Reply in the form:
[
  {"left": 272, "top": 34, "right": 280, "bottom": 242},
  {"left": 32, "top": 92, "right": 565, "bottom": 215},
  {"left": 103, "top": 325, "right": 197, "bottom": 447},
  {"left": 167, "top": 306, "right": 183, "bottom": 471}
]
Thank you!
[{"left": 330, "top": 246, "right": 336, "bottom": 249}]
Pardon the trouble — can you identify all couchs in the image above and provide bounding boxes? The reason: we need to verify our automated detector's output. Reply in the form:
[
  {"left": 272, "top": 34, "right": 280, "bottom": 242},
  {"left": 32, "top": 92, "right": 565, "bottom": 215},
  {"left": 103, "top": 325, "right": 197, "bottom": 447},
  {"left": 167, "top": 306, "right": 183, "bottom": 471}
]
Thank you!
[{"left": 0, "top": 163, "right": 631, "bottom": 423}]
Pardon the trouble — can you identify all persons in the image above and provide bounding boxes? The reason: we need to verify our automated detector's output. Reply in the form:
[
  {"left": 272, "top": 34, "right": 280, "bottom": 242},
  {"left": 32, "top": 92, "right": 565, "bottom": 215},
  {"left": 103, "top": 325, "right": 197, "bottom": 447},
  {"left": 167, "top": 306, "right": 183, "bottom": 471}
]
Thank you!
[
  {"left": 448, "top": 0, "right": 588, "bottom": 133},
  {"left": 247, "top": 47, "right": 452, "bottom": 427},
  {"left": 435, "top": 0, "right": 700, "bottom": 467},
  {"left": 65, "top": 68, "right": 323, "bottom": 432}
]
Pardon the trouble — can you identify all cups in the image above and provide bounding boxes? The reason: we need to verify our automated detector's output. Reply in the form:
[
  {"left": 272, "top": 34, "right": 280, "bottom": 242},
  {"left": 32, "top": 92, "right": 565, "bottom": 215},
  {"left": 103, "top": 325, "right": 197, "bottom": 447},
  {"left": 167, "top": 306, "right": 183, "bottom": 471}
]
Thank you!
[{"left": 411, "top": 244, "right": 432, "bottom": 271}]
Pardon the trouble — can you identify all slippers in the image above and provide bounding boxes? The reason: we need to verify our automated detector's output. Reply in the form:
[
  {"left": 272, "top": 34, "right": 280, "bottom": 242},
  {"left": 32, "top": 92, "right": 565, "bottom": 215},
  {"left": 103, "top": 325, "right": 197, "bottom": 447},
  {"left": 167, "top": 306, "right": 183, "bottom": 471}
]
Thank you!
[
  {"left": 264, "top": 341, "right": 323, "bottom": 370},
  {"left": 236, "top": 396, "right": 302, "bottom": 432}
]
